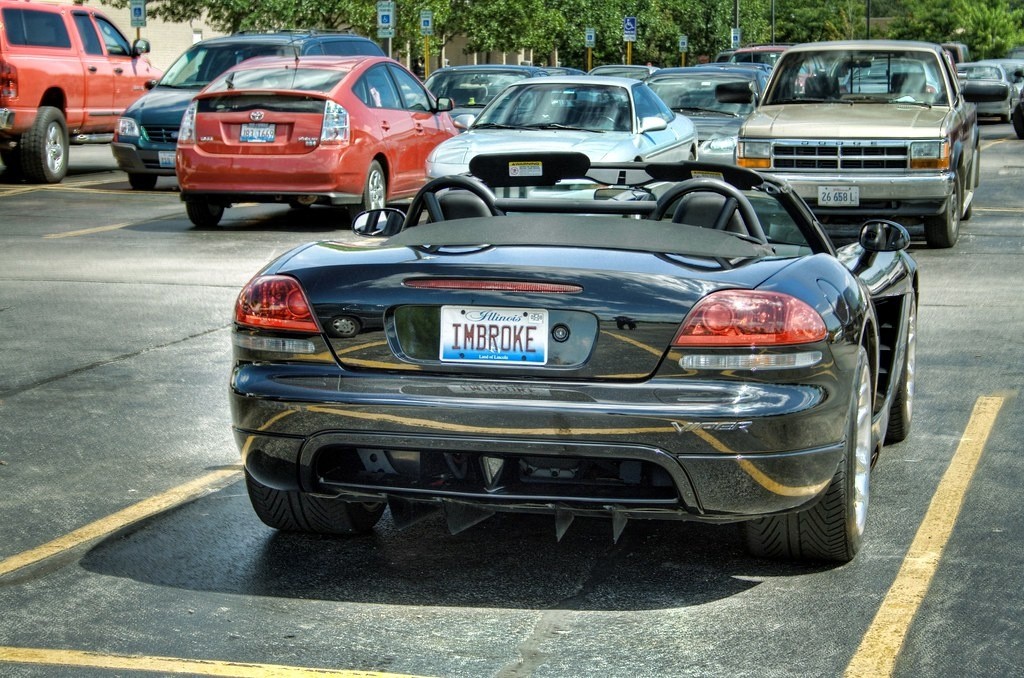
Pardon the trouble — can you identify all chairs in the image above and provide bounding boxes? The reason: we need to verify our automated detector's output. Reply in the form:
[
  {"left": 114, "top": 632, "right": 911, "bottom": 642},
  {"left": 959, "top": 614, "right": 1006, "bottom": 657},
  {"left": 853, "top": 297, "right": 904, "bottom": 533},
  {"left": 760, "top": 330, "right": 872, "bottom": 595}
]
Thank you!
[
  {"left": 672, "top": 191, "right": 750, "bottom": 236},
  {"left": 805, "top": 75, "right": 839, "bottom": 99},
  {"left": 426, "top": 189, "right": 492, "bottom": 225},
  {"left": 891, "top": 72, "right": 927, "bottom": 95}
]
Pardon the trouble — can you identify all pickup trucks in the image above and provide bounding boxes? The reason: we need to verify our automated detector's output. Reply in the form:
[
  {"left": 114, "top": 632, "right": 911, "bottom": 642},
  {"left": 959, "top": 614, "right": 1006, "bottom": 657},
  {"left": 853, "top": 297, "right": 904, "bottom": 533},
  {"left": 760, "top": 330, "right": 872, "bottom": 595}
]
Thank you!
[{"left": 733, "top": 41, "right": 982, "bottom": 246}]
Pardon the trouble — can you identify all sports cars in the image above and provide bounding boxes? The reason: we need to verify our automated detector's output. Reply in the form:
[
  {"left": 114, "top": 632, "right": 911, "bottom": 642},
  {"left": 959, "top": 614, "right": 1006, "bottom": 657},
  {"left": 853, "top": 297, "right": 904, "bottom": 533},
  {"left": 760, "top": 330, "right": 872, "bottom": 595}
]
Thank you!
[{"left": 229, "top": 160, "right": 920, "bottom": 563}]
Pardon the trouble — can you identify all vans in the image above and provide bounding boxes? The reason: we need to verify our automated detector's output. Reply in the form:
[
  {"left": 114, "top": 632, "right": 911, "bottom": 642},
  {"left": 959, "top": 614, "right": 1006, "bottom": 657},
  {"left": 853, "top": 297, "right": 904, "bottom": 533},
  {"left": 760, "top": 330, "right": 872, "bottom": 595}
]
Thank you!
[{"left": 0, "top": 1, "right": 163, "bottom": 183}]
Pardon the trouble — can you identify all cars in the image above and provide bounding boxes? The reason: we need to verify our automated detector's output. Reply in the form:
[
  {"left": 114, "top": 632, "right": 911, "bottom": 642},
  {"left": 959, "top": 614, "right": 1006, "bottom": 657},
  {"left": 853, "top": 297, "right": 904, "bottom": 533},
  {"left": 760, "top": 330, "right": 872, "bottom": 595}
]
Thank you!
[
  {"left": 954, "top": 46, "right": 1024, "bottom": 124},
  {"left": 112, "top": 33, "right": 390, "bottom": 190},
  {"left": 424, "top": 47, "right": 793, "bottom": 165},
  {"left": 176, "top": 57, "right": 461, "bottom": 230},
  {"left": 425, "top": 77, "right": 697, "bottom": 202}
]
